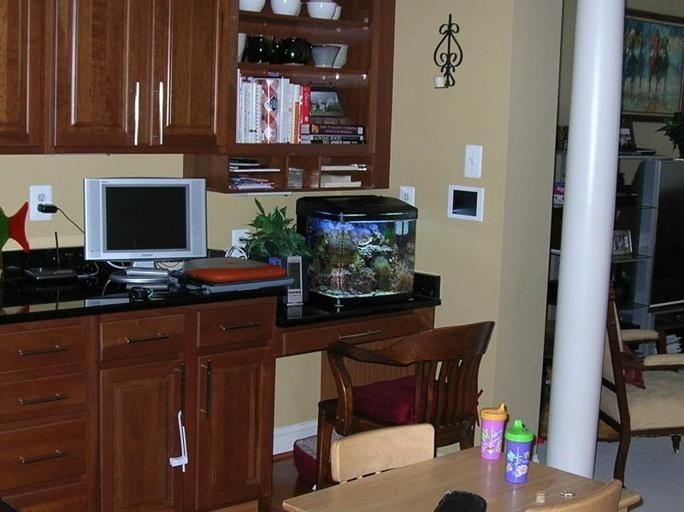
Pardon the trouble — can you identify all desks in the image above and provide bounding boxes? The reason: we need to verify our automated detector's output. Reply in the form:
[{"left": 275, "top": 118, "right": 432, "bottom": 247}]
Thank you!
[
  {"left": 282, "top": 446, "right": 640, "bottom": 512},
  {"left": 276, "top": 283, "right": 442, "bottom": 403}
]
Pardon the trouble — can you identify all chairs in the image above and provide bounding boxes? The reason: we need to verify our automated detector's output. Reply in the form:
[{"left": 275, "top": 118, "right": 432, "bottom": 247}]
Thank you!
[
  {"left": 291, "top": 321, "right": 496, "bottom": 498},
  {"left": 525, "top": 479, "right": 622, "bottom": 512},
  {"left": 330, "top": 424, "right": 435, "bottom": 484},
  {"left": 621, "top": 330, "right": 659, "bottom": 344},
  {"left": 640, "top": 353, "right": 684, "bottom": 371}
]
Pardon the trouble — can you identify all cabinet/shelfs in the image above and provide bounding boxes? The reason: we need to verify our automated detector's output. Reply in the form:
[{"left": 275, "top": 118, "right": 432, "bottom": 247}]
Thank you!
[
  {"left": 52, "top": 0, "right": 227, "bottom": 148},
  {"left": 0, "top": 0, "right": 44, "bottom": 149},
  {"left": 182, "top": 0, "right": 379, "bottom": 195},
  {"left": 99, "top": 294, "right": 275, "bottom": 512},
  {"left": 1, "top": 317, "right": 96, "bottom": 512},
  {"left": 542, "top": 148, "right": 662, "bottom": 357}
]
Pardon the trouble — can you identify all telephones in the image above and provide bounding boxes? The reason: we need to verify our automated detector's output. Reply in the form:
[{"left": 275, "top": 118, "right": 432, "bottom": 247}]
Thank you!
[
  {"left": 287, "top": 255, "right": 304, "bottom": 307},
  {"left": 286, "top": 307, "right": 302, "bottom": 321}
]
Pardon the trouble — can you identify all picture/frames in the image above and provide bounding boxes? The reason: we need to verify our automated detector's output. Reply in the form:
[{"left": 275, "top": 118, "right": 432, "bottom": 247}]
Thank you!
[
  {"left": 619, "top": 7, "right": 684, "bottom": 123},
  {"left": 618, "top": 122, "right": 636, "bottom": 151},
  {"left": 611, "top": 229, "right": 632, "bottom": 255}
]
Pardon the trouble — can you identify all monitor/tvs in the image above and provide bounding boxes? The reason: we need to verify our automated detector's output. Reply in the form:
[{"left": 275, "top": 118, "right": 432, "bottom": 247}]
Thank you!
[{"left": 83, "top": 177, "right": 207, "bottom": 283}]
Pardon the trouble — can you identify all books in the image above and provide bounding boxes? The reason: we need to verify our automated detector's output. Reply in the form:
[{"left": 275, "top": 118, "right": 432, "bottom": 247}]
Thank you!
[
  {"left": 229, "top": 159, "right": 262, "bottom": 169},
  {"left": 237, "top": 68, "right": 367, "bottom": 144},
  {"left": 321, "top": 172, "right": 362, "bottom": 188}
]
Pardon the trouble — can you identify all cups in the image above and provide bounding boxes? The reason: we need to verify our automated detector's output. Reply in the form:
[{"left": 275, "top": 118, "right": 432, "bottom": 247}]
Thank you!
[
  {"left": 505, "top": 419, "right": 535, "bottom": 485},
  {"left": 240, "top": 2, "right": 267, "bottom": 14},
  {"left": 305, "top": 2, "right": 338, "bottom": 19},
  {"left": 270, "top": 0, "right": 301, "bottom": 16},
  {"left": 479, "top": 403, "right": 508, "bottom": 461}
]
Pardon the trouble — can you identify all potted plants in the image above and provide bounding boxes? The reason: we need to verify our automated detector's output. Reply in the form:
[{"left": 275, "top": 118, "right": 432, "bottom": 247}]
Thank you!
[
  {"left": 652, "top": 109, "right": 684, "bottom": 158},
  {"left": 239, "top": 197, "right": 312, "bottom": 304}
]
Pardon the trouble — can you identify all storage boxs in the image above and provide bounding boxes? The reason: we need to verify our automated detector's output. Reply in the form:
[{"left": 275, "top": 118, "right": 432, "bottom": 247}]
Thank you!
[{"left": 599, "top": 299, "right": 684, "bottom": 490}]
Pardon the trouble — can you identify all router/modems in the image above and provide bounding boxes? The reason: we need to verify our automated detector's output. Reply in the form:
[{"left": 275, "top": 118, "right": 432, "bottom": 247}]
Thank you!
[{"left": 25, "top": 232, "right": 77, "bottom": 284}]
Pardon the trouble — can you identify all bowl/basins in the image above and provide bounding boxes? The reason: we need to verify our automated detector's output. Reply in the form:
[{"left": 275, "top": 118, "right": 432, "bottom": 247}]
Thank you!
[
  {"left": 312, "top": 45, "right": 340, "bottom": 67},
  {"left": 238, "top": 33, "right": 245, "bottom": 64},
  {"left": 278, "top": 38, "right": 312, "bottom": 66},
  {"left": 324, "top": 43, "right": 349, "bottom": 69},
  {"left": 247, "top": 34, "right": 275, "bottom": 64}
]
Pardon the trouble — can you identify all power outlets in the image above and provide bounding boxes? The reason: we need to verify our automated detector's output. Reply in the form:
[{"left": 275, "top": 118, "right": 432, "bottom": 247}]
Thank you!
[
  {"left": 231, "top": 228, "right": 251, "bottom": 260},
  {"left": 398, "top": 185, "right": 415, "bottom": 206},
  {"left": 30, "top": 184, "right": 53, "bottom": 221}
]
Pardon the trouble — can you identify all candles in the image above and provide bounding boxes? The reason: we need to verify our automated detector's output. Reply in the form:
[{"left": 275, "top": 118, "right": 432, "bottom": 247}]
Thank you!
[{"left": 433, "top": 75, "right": 446, "bottom": 87}]
